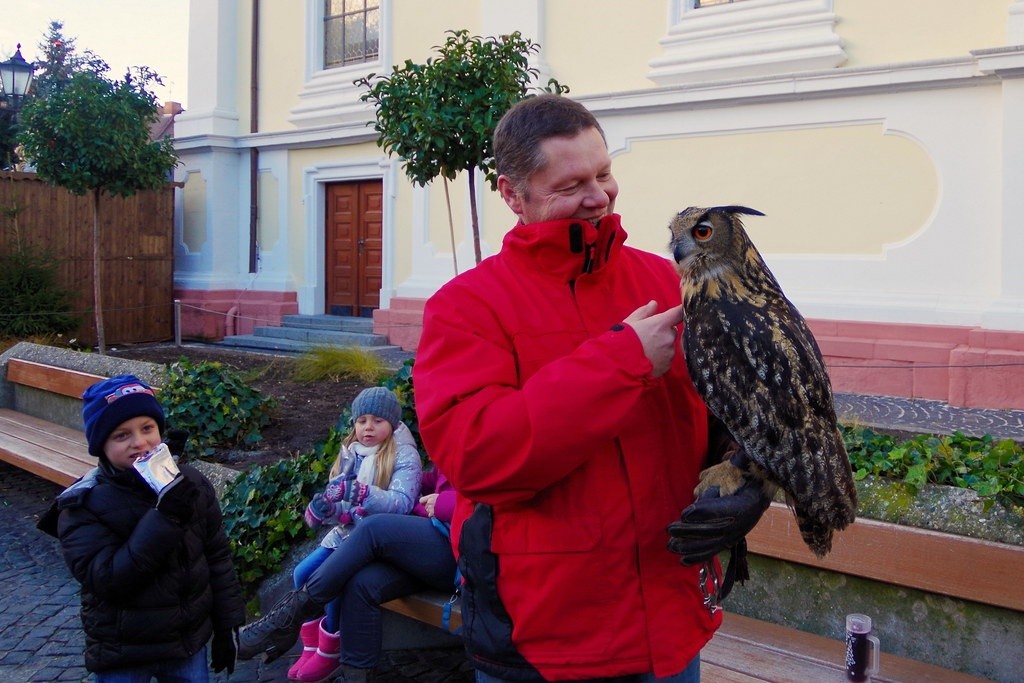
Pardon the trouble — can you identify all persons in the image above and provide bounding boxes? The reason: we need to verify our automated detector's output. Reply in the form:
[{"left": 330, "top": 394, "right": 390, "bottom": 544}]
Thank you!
[
  {"left": 286, "top": 386, "right": 424, "bottom": 683},
  {"left": 412, "top": 94, "right": 782, "bottom": 683},
  {"left": 237, "top": 466, "right": 458, "bottom": 683},
  {"left": 34, "top": 373, "right": 237, "bottom": 683}
]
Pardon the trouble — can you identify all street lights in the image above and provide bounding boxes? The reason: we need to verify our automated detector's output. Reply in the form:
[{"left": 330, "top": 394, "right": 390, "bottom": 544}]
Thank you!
[{"left": 0, "top": 42, "right": 37, "bottom": 124}]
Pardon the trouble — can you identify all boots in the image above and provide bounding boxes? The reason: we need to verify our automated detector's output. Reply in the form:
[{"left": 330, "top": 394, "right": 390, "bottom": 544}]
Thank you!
[
  {"left": 298, "top": 616, "right": 342, "bottom": 683},
  {"left": 287, "top": 610, "right": 328, "bottom": 680},
  {"left": 238, "top": 583, "right": 324, "bottom": 665},
  {"left": 326, "top": 662, "right": 372, "bottom": 683}
]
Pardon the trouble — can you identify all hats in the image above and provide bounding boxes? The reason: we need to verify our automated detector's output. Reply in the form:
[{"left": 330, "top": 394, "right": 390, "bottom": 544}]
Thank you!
[
  {"left": 82, "top": 372, "right": 165, "bottom": 455},
  {"left": 352, "top": 387, "right": 402, "bottom": 432}
]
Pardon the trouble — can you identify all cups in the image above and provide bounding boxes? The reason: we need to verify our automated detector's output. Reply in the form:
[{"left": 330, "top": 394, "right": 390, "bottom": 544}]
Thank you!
[{"left": 844, "top": 614, "right": 880, "bottom": 683}]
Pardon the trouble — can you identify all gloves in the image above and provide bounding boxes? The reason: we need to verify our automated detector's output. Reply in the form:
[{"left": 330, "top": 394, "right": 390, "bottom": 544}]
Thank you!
[
  {"left": 208, "top": 620, "right": 236, "bottom": 677},
  {"left": 326, "top": 479, "right": 369, "bottom": 515},
  {"left": 156, "top": 472, "right": 202, "bottom": 524},
  {"left": 303, "top": 493, "right": 336, "bottom": 532},
  {"left": 667, "top": 476, "right": 773, "bottom": 565}
]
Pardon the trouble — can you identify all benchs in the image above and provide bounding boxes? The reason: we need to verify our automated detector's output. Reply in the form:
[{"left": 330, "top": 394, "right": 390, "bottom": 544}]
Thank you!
[{"left": 0, "top": 349, "right": 1024, "bottom": 683}]
